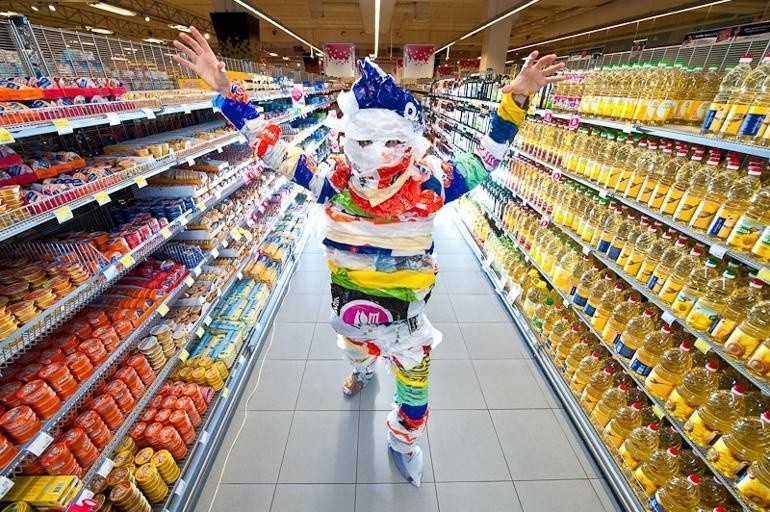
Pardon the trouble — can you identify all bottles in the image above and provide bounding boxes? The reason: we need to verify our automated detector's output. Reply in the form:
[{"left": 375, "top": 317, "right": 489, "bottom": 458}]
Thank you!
[{"left": 431, "top": 53, "right": 770, "bottom": 512}]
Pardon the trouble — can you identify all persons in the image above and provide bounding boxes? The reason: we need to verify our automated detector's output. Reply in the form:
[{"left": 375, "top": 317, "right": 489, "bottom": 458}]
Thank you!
[{"left": 171, "top": 25, "right": 572, "bottom": 488}]
